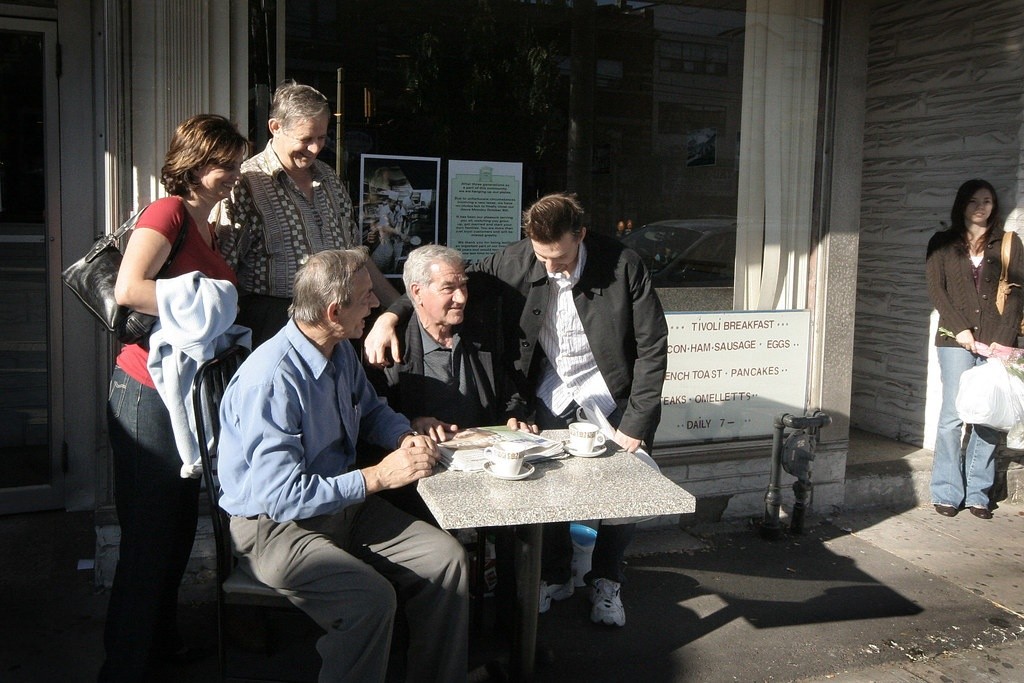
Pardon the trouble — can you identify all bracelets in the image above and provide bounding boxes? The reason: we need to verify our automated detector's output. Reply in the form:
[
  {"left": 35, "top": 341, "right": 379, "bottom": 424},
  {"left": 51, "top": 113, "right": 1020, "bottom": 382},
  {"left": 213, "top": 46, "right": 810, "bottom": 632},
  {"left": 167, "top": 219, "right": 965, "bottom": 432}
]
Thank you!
[{"left": 398, "top": 431, "right": 418, "bottom": 448}]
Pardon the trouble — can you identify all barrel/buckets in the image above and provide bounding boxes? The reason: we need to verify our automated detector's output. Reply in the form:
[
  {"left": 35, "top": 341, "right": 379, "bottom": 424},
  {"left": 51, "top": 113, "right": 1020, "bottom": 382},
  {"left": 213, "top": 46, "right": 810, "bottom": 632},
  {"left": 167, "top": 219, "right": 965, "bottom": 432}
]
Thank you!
[{"left": 569, "top": 524, "right": 597, "bottom": 587}]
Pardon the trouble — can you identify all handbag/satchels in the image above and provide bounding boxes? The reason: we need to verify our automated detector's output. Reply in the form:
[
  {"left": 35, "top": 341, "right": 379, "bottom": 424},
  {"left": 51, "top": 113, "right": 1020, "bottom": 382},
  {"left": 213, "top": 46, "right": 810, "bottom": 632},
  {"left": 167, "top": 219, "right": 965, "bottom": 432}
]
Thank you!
[
  {"left": 60, "top": 201, "right": 187, "bottom": 345},
  {"left": 955, "top": 355, "right": 1016, "bottom": 433},
  {"left": 995, "top": 231, "right": 1024, "bottom": 337}
]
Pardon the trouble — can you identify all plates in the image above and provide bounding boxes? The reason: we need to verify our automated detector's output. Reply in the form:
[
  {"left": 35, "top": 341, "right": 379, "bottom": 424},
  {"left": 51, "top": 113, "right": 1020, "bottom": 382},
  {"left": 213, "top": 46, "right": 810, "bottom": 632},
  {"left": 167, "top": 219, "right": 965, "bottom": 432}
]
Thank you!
[
  {"left": 563, "top": 439, "right": 607, "bottom": 457},
  {"left": 483, "top": 461, "right": 534, "bottom": 480}
]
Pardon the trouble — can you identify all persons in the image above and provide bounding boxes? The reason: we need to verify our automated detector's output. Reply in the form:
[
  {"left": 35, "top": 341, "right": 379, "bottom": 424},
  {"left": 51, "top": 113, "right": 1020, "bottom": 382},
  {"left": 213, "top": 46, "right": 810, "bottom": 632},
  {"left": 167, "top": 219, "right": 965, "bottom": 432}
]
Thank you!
[
  {"left": 105, "top": 114, "right": 248, "bottom": 682},
  {"left": 363, "top": 243, "right": 539, "bottom": 531},
  {"left": 927, "top": 179, "right": 1024, "bottom": 520},
  {"left": 208, "top": 78, "right": 361, "bottom": 351},
  {"left": 364, "top": 192, "right": 669, "bottom": 628},
  {"left": 379, "top": 191, "right": 429, "bottom": 273},
  {"left": 216, "top": 250, "right": 470, "bottom": 682}
]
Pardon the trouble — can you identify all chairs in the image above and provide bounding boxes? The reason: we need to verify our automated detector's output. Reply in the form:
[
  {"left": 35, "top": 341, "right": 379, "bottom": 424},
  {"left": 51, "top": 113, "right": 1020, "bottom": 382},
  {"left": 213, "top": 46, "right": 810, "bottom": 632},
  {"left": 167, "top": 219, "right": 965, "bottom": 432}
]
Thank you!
[{"left": 193, "top": 344, "right": 406, "bottom": 683}]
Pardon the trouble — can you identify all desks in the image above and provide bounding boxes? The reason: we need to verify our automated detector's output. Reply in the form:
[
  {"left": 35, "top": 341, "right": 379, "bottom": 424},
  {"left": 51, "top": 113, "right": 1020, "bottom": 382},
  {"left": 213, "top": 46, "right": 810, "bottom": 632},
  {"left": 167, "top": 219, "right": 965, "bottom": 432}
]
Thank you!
[{"left": 415, "top": 428, "right": 696, "bottom": 683}]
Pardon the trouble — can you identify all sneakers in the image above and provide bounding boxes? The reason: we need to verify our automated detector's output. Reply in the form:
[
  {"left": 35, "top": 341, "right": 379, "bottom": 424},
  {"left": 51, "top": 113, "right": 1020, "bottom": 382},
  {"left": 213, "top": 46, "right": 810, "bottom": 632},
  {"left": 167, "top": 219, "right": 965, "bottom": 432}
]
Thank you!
[
  {"left": 587, "top": 576, "right": 626, "bottom": 626},
  {"left": 539, "top": 575, "right": 574, "bottom": 614}
]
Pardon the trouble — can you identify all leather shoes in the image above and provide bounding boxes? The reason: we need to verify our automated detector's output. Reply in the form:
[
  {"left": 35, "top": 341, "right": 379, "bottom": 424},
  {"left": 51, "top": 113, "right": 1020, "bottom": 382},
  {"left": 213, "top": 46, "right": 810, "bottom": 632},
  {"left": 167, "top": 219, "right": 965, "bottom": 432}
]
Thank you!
[
  {"left": 969, "top": 506, "right": 992, "bottom": 519},
  {"left": 935, "top": 505, "right": 957, "bottom": 517}
]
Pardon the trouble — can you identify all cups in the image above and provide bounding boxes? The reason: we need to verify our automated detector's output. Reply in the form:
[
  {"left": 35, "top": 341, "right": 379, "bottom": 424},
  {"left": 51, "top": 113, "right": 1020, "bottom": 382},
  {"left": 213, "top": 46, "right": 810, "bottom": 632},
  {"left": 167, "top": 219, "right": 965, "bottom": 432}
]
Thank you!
[
  {"left": 569, "top": 423, "right": 605, "bottom": 452},
  {"left": 485, "top": 442, "right": 525, "bottom": 476}
]
